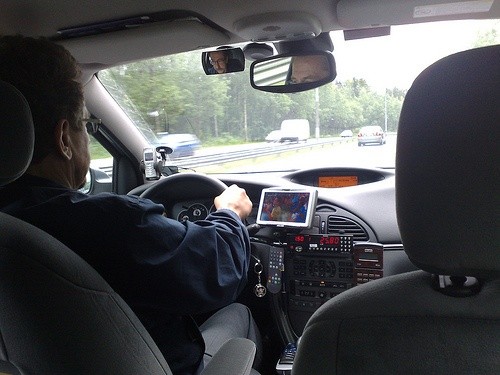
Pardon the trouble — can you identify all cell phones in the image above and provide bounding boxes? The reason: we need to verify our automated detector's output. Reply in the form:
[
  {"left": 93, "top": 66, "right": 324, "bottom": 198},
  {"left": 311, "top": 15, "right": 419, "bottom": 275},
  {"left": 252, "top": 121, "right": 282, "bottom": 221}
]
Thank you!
[{"left": 143, "top": 147, "right": 157, "bottom": 178}]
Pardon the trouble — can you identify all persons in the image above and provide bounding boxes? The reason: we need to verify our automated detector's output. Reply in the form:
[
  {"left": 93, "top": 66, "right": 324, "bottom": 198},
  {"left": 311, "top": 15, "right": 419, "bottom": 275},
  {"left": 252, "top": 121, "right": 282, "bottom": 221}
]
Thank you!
[
  {"left": 260, "top": 192, "right": 309, "bottom": 223},
  {"left": 0, "top": 33, "right": 280, "bottom": 375},
  {"left": 288, "top": 54, "right": 331, "bottom": 85},
  {"left": 207, "top": 51, "right": 241, "bottom": 74}
]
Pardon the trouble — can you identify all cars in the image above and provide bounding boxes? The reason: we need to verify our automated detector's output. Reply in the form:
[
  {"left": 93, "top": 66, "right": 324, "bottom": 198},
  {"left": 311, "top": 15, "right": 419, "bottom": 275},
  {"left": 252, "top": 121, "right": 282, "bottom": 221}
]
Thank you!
[
  {"left": 358, "top": 126, "right": 386, "bottom": 147},
  {"left": 340, "top": 130, "right": 354, "bottom": 137},
  {"left": 264, "top": 130, "right": 280, "bottom": 142},
  {"left": 152, "top": 133, "right": 200, "bottom": 159}
]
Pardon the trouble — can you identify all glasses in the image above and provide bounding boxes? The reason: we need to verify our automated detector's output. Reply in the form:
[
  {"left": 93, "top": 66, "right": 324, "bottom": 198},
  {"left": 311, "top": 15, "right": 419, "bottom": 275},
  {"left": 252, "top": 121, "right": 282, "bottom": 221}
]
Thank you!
[
  {"left": 210, "top": 59, "right": 226, "bottom": 64},
  {"left": 288, "top": 78, "right": 324, "bottom": 84},
  {"left": 65, "top": 117, "right": 101, "bottom": 134}
]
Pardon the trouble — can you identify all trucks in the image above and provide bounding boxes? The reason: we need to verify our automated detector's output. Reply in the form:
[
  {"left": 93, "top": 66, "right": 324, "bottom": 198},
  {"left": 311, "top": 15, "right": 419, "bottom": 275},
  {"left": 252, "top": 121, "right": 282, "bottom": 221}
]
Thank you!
[{"left": 280, "top": 119, "right": 310, "bottom": 143}]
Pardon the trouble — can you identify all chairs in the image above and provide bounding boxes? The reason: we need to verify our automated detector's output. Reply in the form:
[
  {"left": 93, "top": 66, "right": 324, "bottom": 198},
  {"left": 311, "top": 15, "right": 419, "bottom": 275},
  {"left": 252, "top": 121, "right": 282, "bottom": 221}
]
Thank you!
[
  {"left": 289, "top": 43, "right": 500, "bottom": 375},
  {"left": 0, "top": 83, "right": 262, "bottom": 375}
]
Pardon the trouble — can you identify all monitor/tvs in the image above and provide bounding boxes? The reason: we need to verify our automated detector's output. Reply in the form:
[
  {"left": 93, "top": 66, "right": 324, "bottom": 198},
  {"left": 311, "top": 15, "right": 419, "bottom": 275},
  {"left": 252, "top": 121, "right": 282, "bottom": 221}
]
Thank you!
[{"left": 256, "top": 187, "right": 318, "bottom": 229}]
